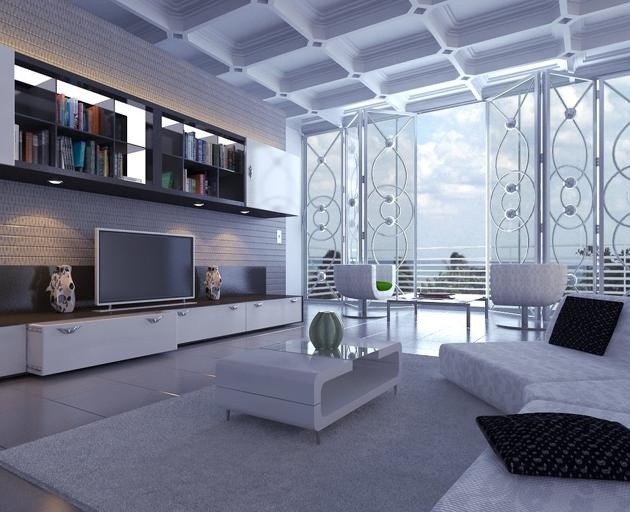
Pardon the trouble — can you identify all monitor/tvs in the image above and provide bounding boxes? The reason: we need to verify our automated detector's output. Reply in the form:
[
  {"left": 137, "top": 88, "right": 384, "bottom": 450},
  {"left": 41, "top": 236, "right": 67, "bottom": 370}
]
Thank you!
[{"left": 92, "top": 226, "right": 199, "bottom": 312}]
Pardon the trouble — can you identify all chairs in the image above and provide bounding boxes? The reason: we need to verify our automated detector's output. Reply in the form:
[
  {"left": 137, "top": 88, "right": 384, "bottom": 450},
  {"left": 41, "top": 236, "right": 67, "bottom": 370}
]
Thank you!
[
  {"left": 490, "top": 261, "right": 568, "bottom": 341},
  {"left": 332, "top": 262, "right": 397, "bottom": 320},
  {"left": 523, "top": 379, "right": 629, "bottom": 412}
]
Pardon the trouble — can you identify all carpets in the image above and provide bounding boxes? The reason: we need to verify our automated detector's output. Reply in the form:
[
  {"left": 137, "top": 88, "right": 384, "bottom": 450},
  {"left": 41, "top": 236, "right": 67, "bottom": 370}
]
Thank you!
[{"left": 0, "top": 352, "right": 506, "bottom": 511}]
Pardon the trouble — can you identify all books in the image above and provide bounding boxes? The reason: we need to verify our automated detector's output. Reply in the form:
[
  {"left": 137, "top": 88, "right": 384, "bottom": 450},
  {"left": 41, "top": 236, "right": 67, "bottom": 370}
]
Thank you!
[
  {"left": 184, "top": 131, "right": 247, "bottom": 200},
  {"left": 14, "top": 92, "right": 126, "bottom": 178}
]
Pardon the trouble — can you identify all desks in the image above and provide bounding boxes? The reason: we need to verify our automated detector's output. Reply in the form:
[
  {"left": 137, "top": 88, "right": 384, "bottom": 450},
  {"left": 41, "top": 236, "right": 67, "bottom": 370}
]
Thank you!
[{"left": 386, "top": 291, "right": 489, "bottom": 343}]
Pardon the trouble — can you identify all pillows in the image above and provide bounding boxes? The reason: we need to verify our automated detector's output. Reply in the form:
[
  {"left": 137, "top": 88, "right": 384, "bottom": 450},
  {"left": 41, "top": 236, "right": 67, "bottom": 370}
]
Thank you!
[
  {"left": 375, "top": 280, "right": 391, "bottom": 290},
  {"left": 548, "top": 295, "right": 625, "bottom": 356},
  {"left": 471, "top": 412, "right": 630, "bottom": 482}
]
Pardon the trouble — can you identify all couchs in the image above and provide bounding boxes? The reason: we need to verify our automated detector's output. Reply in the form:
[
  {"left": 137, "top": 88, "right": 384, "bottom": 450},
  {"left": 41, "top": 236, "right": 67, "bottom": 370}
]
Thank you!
[
  {"left": 431, "top": 400, "right": 630, "bottom": 511},
  {"left": 439, "top": 294, "right": 630, "bottom": 414}
]
